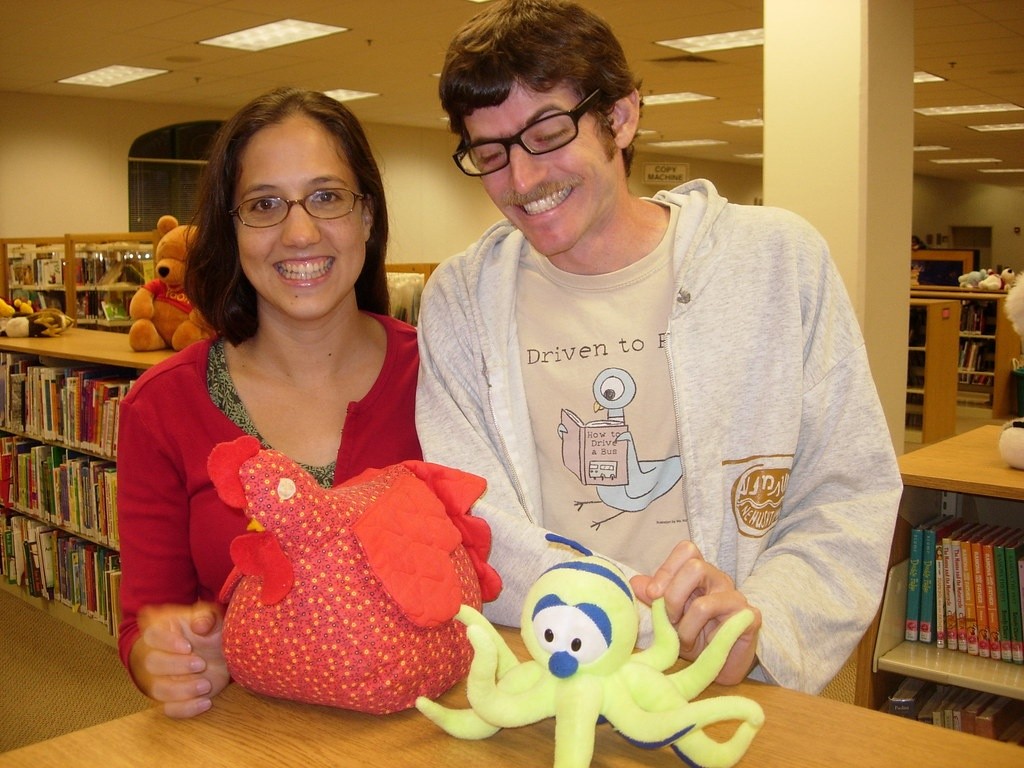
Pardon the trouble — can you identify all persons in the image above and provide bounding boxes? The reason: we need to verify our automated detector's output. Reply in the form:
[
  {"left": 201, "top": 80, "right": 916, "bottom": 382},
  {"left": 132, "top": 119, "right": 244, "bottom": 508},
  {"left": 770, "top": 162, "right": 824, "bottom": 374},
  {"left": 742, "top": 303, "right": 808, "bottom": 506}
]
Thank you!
[
  {"left": 414, "top": 0, "right": 905, "bottom": 695},
  {"left": 117, "top": 88, "right": 424, "bottom": 719}
]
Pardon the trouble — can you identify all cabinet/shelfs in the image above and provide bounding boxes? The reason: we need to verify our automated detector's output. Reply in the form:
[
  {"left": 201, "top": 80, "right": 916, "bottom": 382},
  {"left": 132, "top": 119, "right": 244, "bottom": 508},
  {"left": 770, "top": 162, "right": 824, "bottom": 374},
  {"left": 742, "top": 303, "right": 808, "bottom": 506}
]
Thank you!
[
  {"left": 854, "top": 424, "right": 1024, "bottom": 746},
  {"left": 905, "top": 282, "right": 1024, "bottom": 447},
  {"left": 0, "top": 230, "right": 162, "bottom": 334},
  {"left": 0, "top": 323, "right": 183, "bottom": 651}
]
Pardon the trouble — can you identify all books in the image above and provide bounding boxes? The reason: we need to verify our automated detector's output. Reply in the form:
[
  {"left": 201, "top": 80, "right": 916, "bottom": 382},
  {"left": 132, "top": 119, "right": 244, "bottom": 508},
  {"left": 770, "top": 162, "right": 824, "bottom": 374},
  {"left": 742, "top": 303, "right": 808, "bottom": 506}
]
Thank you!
[
  {"left": 907, "top": 301, "right": 992, "bottom": 432},
  {"left": 0, "top": 352, "right": 137, "bottom": 638},
  {"left": 76, "top": 242, "right": 155, "bottom": 322},
  {"left": 886, "top": 514, "right": 1024, "bottom": 746},
  {"left": 7, "top": 242, "right": 65, "bottom": 315}
]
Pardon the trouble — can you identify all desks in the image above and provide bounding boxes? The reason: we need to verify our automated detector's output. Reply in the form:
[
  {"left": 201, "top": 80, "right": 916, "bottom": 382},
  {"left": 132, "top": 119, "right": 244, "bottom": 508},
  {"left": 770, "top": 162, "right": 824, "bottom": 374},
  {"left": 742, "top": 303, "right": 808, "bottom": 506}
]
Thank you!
[{"left": 0, "top": 614, "right": 1024, "bottom": 768}]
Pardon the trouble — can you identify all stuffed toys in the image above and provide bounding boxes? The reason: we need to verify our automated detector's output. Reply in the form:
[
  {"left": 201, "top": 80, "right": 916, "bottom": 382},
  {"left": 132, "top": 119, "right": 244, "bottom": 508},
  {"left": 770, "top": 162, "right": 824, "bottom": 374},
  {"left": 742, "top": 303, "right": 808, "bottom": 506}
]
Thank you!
[
  {"left": 957, "top": 266, "right": 1016, "bottom": 290},
  {"left": 0, "top": 308, "right": 72, "bottom": 337},
  {"left": 129, "top": 215, "right": 217, "bottom": 352},
  {"left": 0, "top": 298, "right": 33, "bottom": 316},
  {"left": 206, "top": 435, "right": 502, "bottom": 716},
  {"left": 415, "top": 533, "right": 765, "bottom": 768},
  {"left": 998, "top": 272, "right": 1024, "bottom": 474}
]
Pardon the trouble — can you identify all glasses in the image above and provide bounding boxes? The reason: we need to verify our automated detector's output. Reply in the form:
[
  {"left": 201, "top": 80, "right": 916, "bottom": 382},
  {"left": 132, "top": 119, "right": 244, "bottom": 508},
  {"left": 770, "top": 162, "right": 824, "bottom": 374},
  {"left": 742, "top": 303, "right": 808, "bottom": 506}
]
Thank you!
[
  {"left": 228, "top": 187, "right": 364, "bottom": 228},
  {"left": 452, "top": 87, "right": 601, "bottom": 177}
]
